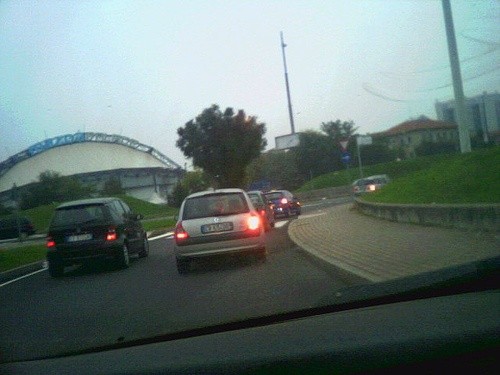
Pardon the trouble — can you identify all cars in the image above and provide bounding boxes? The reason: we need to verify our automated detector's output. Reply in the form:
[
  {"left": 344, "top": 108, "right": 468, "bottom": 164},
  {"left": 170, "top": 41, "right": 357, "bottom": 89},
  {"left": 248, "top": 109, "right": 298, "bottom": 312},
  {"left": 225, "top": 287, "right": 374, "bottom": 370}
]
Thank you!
[
  {"left": 247, "top": 191, "right": 275, "bottom": 230},
  {"left": 173, "top": 189, "right": 268, "bottom": 274},
  {"left": 352, "top": 175, "right": 389, "bottom": 196},
  {"left": 265, "top": 190, "right": 302, "bottom": 219},
  {"left": 0, "top": 216, "right": 37, "bottom": 242},
  {"left": 46, "top": 197, "right": 150, "bottom": 277}
]
